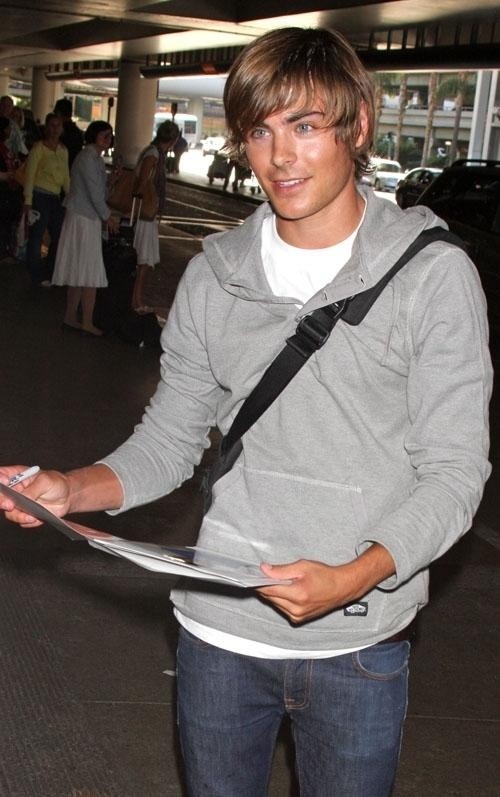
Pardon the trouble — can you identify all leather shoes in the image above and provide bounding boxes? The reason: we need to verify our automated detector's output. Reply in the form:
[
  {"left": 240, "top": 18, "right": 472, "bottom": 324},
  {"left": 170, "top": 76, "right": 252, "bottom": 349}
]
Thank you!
[{"left": 61, "top": 315, "right": 112, "bottom": 340}]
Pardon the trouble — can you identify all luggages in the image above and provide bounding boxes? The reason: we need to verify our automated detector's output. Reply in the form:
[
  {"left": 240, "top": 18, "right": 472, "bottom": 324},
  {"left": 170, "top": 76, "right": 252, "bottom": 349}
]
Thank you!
[{"left": 98, "top": 194, "right": 143, "bottom": 307}]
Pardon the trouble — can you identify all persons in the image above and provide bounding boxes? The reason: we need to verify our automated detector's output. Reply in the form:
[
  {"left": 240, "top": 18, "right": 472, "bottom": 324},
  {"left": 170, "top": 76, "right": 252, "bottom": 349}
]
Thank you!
[
  {"left": 106, "top": 154, "right": 132, "bottom": 235},
  {"left": 1, "top": 23, "right": 496, "bottom": 797},
  {"left": 51, "top": 121, "right": 118, "bottom": 339},
  {"left": 2, "top": 96, "right": 84, "bottom": 288},
  {"left": 132, "top": 120, "right": 180, "bottom": 313},
  {"left": 232, "top": 159, "right": 253, "bottom": 192}
]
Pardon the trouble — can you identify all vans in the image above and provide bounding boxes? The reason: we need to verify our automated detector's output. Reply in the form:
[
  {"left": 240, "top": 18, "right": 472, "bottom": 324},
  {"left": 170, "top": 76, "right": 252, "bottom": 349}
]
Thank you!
[{"left": 152, "top": 111, "right": 199, "bottom": 148}]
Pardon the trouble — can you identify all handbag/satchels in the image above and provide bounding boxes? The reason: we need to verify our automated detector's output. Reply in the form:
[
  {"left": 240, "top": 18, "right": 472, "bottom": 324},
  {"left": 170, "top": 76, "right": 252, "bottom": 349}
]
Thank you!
[
  {"left": 104, "top": 144, "right": 161, "bottom": 223},
  {"left": 11, "top": 205, "right": 30, "bottom": 262}
]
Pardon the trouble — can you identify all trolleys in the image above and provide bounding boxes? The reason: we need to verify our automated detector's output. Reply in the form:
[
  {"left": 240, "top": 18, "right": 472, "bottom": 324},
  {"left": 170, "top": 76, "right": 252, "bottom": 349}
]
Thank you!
[{"left": 207, "top": 149, "right": 235, "bottom": 190}]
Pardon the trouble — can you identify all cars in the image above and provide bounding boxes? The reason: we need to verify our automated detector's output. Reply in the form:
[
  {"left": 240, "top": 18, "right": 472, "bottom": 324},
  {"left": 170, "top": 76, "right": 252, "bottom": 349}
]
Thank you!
[
  {"left": 357, "top": 158, "right": 500, "bottom": 277},
  {"left": 200, "top": 136, "right": 226, "bottom": 156}
]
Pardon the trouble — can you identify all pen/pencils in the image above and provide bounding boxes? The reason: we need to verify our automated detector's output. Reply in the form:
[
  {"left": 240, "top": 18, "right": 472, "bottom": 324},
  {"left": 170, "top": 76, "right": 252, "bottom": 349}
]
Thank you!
[{"left": 8, "top": 466, "right": 40, "bottom": 488}]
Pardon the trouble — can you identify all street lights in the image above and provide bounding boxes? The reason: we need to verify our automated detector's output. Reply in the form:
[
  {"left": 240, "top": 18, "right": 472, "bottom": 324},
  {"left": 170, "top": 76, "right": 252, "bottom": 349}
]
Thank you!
[
  {"left": 107, "top": 96, "right": 114, "bottom": 123},
  {"left": 171, "top": 101, "right": 178, "bottom": 122}
]
